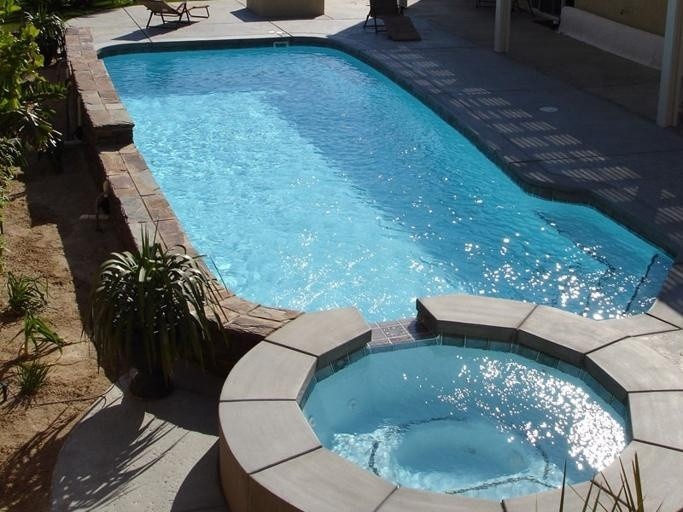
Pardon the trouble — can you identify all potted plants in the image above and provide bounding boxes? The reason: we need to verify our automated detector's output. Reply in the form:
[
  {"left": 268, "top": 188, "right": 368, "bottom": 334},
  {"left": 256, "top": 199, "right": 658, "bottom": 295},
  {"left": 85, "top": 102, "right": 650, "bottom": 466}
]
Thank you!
[{"left": 77, "top": 216, "right": 230, "bottom": 400}]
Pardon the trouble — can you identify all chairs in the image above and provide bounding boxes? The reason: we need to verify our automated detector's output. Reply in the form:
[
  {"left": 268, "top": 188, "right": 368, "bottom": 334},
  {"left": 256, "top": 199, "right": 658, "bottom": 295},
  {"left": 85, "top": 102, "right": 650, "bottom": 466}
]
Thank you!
[
  {"left": 363, "top": 0, "right": 422, "bottom": 42},
  {"left": 141, "top": 0, "right": 210, "bottom": 31}
]
